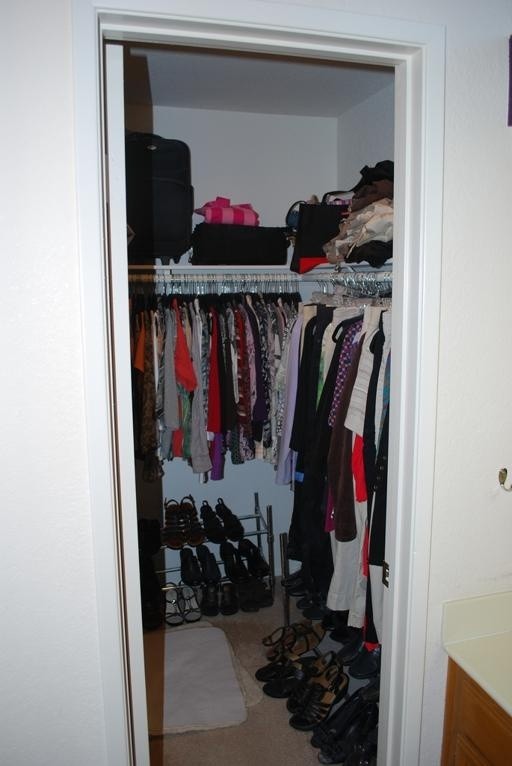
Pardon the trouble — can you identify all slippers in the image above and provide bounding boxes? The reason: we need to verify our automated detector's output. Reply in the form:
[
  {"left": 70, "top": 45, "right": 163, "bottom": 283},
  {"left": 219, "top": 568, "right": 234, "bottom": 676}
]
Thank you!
[
  {"left": 196, "top": 545, "right": 221, "bottom": 585},
  {"left": 180, "top": 548, "right": 202, "bottom": 586}
]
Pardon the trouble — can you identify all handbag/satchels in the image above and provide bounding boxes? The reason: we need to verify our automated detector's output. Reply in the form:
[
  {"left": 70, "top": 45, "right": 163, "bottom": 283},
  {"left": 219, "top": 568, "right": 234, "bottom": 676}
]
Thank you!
[
  {"left": 290, "top": 204, "right": 350, "bottom": 273},
  {"left": 190, "top": 224, "right": 291, "bottom": 266}
]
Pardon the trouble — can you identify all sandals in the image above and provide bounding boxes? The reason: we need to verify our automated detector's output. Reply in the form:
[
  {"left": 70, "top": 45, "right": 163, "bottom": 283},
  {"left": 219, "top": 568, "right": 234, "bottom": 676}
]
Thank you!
[
  {"left": 215, "top": 498, "right": 244, "bottom": 541},
  {"left": 180, "top": 494, "right": 204, "bottom": 547},
  {"left": 220, "top": 541, "right": 248, "bottom": 584},
  {"left": 164, "top": 497, "right": 184, "bottom": 550},
  {"left": 256, "top": 619, "right": 378, "bottom": 766},
  {"left": 179, "top": 580, "right": 201, "bottom": 621},
  {"left": 238, "top": 539, "right": 268, "bottom": 577},
  {"left": 199, "top": 500, "right": 225, "bottom": 544},
  {"left": 200, "top": 584, "right": 218, "bottom": 616},
  {"left": 220, "top": 584, "right": 237, "bottom": 615},
  {"left": 161, "top": 582, "right": 184, "bottom": 625}
]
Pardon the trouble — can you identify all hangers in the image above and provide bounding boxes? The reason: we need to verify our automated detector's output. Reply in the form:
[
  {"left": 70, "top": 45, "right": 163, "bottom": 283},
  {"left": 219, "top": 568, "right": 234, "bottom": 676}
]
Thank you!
[{"left": 128, "top": 271, "right": 393, "bottom": 351}]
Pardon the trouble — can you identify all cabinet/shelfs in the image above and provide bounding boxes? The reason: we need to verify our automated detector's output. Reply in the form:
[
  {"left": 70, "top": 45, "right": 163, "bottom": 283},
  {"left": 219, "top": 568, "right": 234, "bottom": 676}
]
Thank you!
[
  {"left": 278, "top": 531, "right": 381, "bottom": 741},
  {"left": 153, "top": 493, "right": 274, "bottom": 626}
]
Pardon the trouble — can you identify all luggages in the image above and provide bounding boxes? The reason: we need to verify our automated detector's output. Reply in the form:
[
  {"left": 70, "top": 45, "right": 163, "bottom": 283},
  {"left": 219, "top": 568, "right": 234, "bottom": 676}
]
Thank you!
[{"left": 125, "top": 133, "right": 193, "bottom": 264}]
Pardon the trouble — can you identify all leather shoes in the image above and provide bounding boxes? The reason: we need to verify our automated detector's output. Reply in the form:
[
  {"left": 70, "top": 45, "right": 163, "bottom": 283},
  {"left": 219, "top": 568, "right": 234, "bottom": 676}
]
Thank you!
[{"left": 281, "top": 569, "right": 381, "bottom": 679}]
